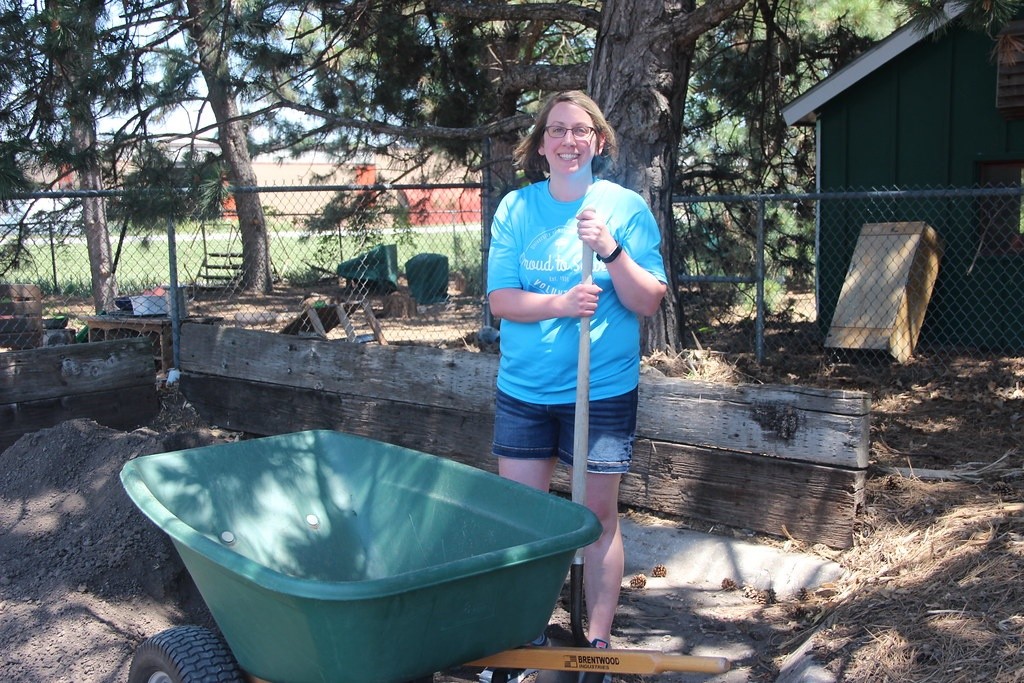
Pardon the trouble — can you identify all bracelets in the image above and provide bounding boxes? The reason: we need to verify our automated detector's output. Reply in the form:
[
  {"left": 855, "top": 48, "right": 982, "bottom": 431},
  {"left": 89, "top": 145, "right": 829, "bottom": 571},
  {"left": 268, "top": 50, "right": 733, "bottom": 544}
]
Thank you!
[{"left": 596, "top": 243, "right": 623, "bottom": 263}]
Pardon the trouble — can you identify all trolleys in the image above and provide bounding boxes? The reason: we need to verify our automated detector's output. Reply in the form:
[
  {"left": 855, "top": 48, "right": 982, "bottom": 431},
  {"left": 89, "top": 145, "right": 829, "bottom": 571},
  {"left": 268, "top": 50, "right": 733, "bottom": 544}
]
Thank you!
[
  {"left": 123, "top": 429, "right": 734, "bottom": 683},
  {"left": 304, "top": 242, "right": 401, "bottom": 294}
]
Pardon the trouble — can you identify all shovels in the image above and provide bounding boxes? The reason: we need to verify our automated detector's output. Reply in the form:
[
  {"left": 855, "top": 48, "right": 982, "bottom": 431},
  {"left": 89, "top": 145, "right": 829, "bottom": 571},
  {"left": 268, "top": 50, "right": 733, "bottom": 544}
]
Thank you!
[{"left": 525, "top": 207, "right": 631, "bottom": 683}]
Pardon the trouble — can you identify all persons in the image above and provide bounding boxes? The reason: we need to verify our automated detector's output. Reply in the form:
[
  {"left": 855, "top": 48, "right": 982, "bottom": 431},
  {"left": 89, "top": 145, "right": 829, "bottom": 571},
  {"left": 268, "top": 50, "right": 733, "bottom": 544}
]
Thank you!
[{"left": 486, "top": 91, "right": 668, "bottom": 683}]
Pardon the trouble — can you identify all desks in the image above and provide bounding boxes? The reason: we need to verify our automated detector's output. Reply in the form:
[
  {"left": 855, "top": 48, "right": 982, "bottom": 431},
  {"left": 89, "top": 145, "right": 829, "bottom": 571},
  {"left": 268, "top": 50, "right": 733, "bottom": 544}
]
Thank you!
[{"left": 87, "top": 314, "right": 224, "bottom": 373}]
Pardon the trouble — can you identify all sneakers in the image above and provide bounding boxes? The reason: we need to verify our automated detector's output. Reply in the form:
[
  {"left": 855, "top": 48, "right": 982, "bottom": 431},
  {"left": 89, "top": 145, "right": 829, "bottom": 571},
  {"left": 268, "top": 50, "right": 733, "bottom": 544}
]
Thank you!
[
  {"left": 477, "top": 632, "right": 552, "bottom": 683},
  {"left": 577, "top": 639, "right": 613, "bottom": 683}
]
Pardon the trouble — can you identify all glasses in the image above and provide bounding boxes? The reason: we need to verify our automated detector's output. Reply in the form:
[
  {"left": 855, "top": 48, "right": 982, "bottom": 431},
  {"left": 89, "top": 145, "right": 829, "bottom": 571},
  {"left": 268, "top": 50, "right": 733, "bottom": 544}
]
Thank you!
[{"left": 544, "top": 125, "right": 598, "bottom": 141}]
